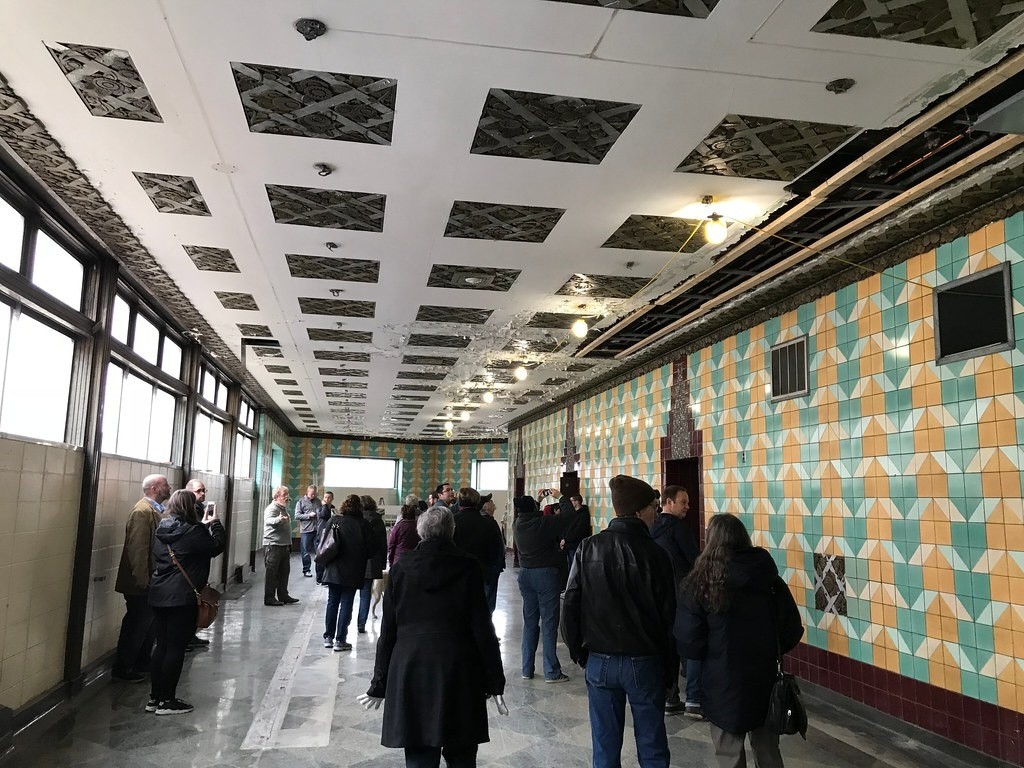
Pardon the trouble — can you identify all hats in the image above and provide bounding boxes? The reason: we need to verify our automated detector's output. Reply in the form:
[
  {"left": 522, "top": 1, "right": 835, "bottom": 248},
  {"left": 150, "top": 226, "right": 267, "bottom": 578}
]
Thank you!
[
  {"left": 609, "top": 474, "right": 657, "bottom": 517},
  {"left": 479, "top": 492, "right": 493, "bottom": 511}
]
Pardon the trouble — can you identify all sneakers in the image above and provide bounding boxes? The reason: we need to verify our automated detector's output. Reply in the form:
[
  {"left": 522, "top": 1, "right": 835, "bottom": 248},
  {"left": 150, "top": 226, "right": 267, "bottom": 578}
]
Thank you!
[
  {"left": 145, "top": 698, "right": 159, "bottom": 712},
  {"left": 154, "top": 698, "right": 194, "bottom": 714}
]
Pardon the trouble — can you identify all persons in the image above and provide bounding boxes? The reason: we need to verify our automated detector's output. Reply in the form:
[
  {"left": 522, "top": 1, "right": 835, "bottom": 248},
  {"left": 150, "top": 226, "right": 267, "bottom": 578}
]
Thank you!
[
  {"left": 388, "top": 483, "right": 505, "bottom": 612},
  {"left": 183, "top": 479, "right": 210, "bottom": 651},
  {"left": 560, "top": 475, "right": 678, "bottom": 768},
  {"left": 653, "top": 485, "right": 706, "bottom": 719},
  {"left": 358, "top": 508, "right": 508, "bottom": 768},
  {"left": 514, "top": 490, "right": 591, "bottom": 682},
  {"left": 110, "top": 474, "right": 172, "bottom": 683},
  {"left": 145, "top": 490, "right": 226, "bottom": 715},
  {"left": 315, "top": 495, "right": 387, "bottom": 650},
  {"left": 675, "top": 514, "right": 804, "bottom": 768},
  {"left": 264, "top": 487, "right": 299, "bottom": 606},
  {"left": 295, "top": 485, "right": 335, "bottom": 578}
]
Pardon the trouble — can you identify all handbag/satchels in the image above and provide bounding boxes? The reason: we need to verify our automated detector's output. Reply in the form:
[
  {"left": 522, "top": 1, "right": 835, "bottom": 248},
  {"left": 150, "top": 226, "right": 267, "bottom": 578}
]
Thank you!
[
  {"left": 196, "top": 586, "right": 221, "bottom": 628},
  {"left": 769, "top": 659, "right": 808, "bottom": 740},
  {"left": 314, "top": 516, "right": 339, "bottom": 565}
]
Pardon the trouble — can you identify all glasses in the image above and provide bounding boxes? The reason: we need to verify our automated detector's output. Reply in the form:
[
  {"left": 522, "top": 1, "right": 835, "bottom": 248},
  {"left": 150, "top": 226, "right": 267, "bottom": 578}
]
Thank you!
[
  {"left": 647, "top": 502, "right": 658, "bottom": 513},
  {"left": 192, "top": 488, "right": 209, "bottom": 494}
]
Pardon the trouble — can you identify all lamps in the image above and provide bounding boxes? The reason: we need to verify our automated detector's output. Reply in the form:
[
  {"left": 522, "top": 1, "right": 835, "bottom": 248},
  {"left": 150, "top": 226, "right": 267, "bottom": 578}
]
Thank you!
[
  {"left": 572, "top": 318, "right": 588, "bottom": 338},
  {"left": 445, "top": 420, "right": 454, "bottom": 430},
  {"left": 515, "top": 366, "right": 527, "bottom": 379},
  {"left": 704, "top": 212, "right": 728, "bottom": 244},
  {"left": 461, "top": 408, "right": 470, "bottom": 421},
  {"left": 483, "top": 391, "right": 493, "bottom": 402}
]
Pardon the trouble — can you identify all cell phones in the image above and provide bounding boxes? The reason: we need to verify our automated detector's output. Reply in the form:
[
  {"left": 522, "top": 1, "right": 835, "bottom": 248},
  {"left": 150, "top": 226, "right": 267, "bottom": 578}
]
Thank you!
[
  {"left": 207, "top": 502, "right": 215, "bottom": 520},
  {"left": 543, "top": 489, "right": 551, "bottom": 496}
]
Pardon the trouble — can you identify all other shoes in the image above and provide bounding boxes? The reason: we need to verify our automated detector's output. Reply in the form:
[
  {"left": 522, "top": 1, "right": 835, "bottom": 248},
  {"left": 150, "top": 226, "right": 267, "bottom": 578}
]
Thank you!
[
  {"left": 522, "top": 673, "right": 534, "bottom": 679},
  {"left": 111, "top": 672, "right": 144, "bottom": 683},
  {"left": 304, "top": 571, "right": 312, "bottom": 577},
  {"left": 278, "top": 595, "right": 299, "bottom": 604},
  {"left": 665, "top": 701, "right": 685, "bottom": 716},
  {"left": 334, "top": 641, "right": 352, "bottom": 650},
  {"left": 184, "top": 645, "right": 196, "bottom": 654},
  {"left": 323, "top": 635, "right": 333, "bottom": 648},
  {"left": 264, "top": 597, "right": 285, "bottom": 606},
  {"left": 546, "top": 672, "right": 569, "bottom": 683},
  {"left": 187, "top": 636, "right": 210, "bottom": 647},
  {"left": 683, "top": 703, "right": 707, "bottom": 718},
  {"left": 359, "top": 628, "right": 365, "bottom": 633}
]
